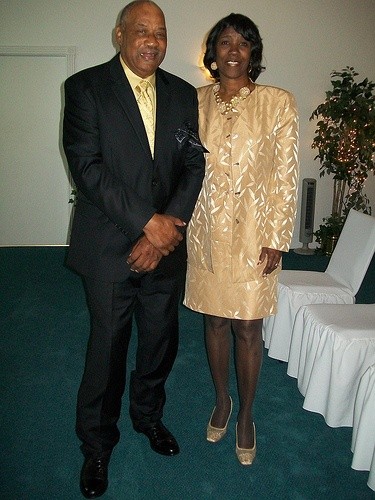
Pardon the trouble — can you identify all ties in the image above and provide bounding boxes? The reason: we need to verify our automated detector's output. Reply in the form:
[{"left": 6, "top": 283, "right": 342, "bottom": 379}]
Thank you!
[{"left": 136, "top": 81, "right": 155, "bottom": 157}]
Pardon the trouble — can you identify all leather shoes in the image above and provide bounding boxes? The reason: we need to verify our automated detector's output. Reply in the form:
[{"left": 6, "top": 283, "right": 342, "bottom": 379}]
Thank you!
[
  {"left": 133, "top": 419, "right": 180, "bottom": 456},
  {"left": 206, "top": 394, "right": 232, "bottom": 442},
  {"left": 234, "top": 421, "right": 257, "bottom": 465},
  {"left": 80, "top": 448, "right": 113, "bottom": 499}
]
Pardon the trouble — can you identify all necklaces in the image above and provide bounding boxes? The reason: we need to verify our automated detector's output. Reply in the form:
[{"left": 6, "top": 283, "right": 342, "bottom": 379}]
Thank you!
[{"left": 213, "top": 82, "right": 251, "bottom": 115}]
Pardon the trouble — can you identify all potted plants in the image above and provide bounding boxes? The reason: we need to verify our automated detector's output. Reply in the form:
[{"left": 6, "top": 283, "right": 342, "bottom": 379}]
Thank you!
[{"left": 308, "top": 65, "right": 375, "bottom": 256}]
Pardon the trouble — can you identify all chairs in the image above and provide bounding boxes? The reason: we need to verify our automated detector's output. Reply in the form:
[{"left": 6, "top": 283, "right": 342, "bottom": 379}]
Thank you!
[
  {"left": 262, "top": 209, "right": 375, "bottom": 361},
  {"left": 286, "top": 304, "right": 375, "bottom": 492}
]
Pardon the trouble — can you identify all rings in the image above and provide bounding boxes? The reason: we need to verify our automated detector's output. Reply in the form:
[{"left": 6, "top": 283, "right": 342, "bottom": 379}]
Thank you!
[{"left": 275, "top": 264, "right": 279, "bottom": 267}]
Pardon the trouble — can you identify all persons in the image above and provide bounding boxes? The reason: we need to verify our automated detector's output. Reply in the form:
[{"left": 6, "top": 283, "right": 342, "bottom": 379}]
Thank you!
[
  {"left": 182, "top": 14, "right": 299, "bottom": 465},
  {"left": 63, "top": 0, "right": 206, "bottom": 500}
]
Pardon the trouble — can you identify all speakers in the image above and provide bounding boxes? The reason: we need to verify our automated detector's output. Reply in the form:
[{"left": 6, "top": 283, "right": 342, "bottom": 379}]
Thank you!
[{"left": 294, "top": 178, "right": 317, "bottom": 255}]
